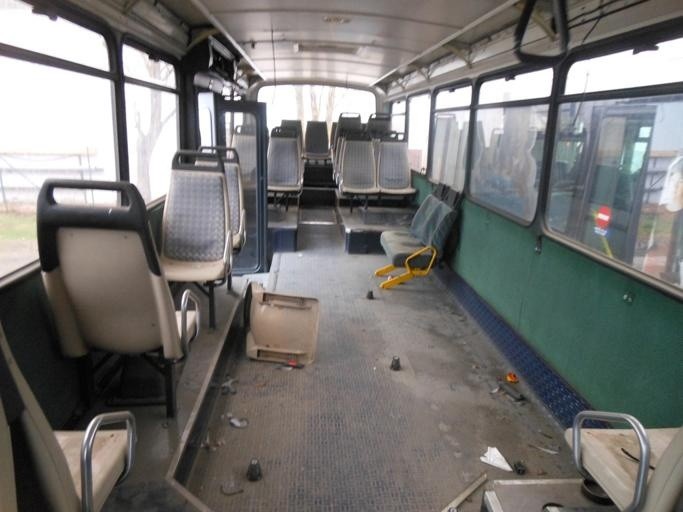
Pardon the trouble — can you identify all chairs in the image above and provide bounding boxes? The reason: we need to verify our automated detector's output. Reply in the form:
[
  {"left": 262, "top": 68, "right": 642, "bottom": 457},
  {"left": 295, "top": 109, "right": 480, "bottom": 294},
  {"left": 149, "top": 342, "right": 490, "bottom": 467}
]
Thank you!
[
  {"left": 303, "top": 119, "right": 333, "bottom": 170},
  {"left": 160, "top": 149, "right": 234, "bottom": 328},
  {"left": 374, "top": 194, "right": 456, "bottom": 291},
  {"left": 564, "top": 410, "right": 683, "bottom": 512},
  {"left": 194, "top": 144, "right": 246, "bottom": 295},
  {"left": 244, "top": 279, "right": 323, "bottom": 370},
  {"left": 330, "top": 112, "right": 419, "bottom": 214},
  {"left": 35, "top": 175, "right": 205, "bottom": 423},
  {"left": 226, "top": 116, "right": 304, "bottom": 212},
  {"left": 0, "top": 319, "right": 139, "bottom": 512}
]
